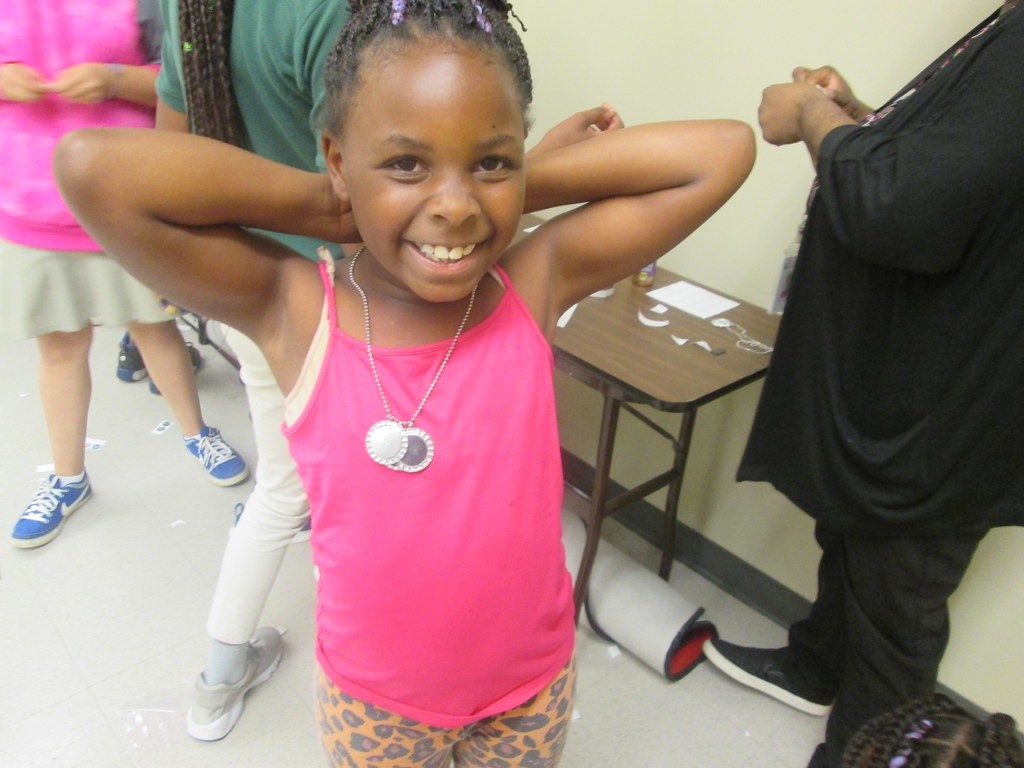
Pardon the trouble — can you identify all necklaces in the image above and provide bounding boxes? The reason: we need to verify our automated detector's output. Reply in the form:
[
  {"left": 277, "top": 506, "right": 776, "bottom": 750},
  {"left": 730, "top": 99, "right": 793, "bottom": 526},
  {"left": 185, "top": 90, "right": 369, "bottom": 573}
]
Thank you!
[{"left": 349, "top": 246, "right": 479, "bottom": 473}]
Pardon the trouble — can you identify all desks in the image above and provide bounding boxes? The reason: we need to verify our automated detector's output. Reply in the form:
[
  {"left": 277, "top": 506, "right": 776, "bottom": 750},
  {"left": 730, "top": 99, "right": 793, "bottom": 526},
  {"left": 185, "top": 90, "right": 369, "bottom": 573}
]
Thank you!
[{"left": 197, "top": 215, "right": 782, "bottom": 628}]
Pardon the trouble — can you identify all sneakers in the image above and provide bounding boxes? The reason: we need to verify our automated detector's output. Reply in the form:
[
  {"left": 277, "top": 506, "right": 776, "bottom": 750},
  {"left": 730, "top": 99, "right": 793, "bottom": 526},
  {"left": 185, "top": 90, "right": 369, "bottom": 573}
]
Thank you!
[
  {"left": 703, "top": 638, "right": 837, "bottom": 717},
  {"left": 116, "top": 331, "right": 148, "bottom": 381},
  {"left": 150, "top": 341, "right": 200, "bottom": 394},
  {"left": 183, "top": 426, "right": 249, "bottom": 486},
  {"left": 10, "top": 471, "right": 93, "bottom": 548},
  {"left": 186, "top": 626, "right": 282, "bottom": 741}
]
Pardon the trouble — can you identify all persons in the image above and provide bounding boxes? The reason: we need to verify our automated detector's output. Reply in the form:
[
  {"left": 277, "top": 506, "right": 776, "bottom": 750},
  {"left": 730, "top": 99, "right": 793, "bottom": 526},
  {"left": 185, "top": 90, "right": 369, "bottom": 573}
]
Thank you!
[
  {"left": 0, "top": 1, "right": 249, "bottom": 547},
  {"left": 53, "top": 1, "right": 757, "bottom": 768},
  {"left": 151, "top": 0, "right": 356, "bottom": 741},
  {"left": 839, "top": 691, "right": 1024, "bottom": 768},
  {"left": 699, "top": 0, "right": 1024, "bottom": 768}
]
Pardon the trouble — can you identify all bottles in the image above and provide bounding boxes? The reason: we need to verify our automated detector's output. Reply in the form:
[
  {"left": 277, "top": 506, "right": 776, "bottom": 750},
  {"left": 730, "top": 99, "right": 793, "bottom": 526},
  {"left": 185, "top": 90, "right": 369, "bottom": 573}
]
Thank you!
[{"left": 633, "top": 261, "right": 656, "bottom": 286}]
